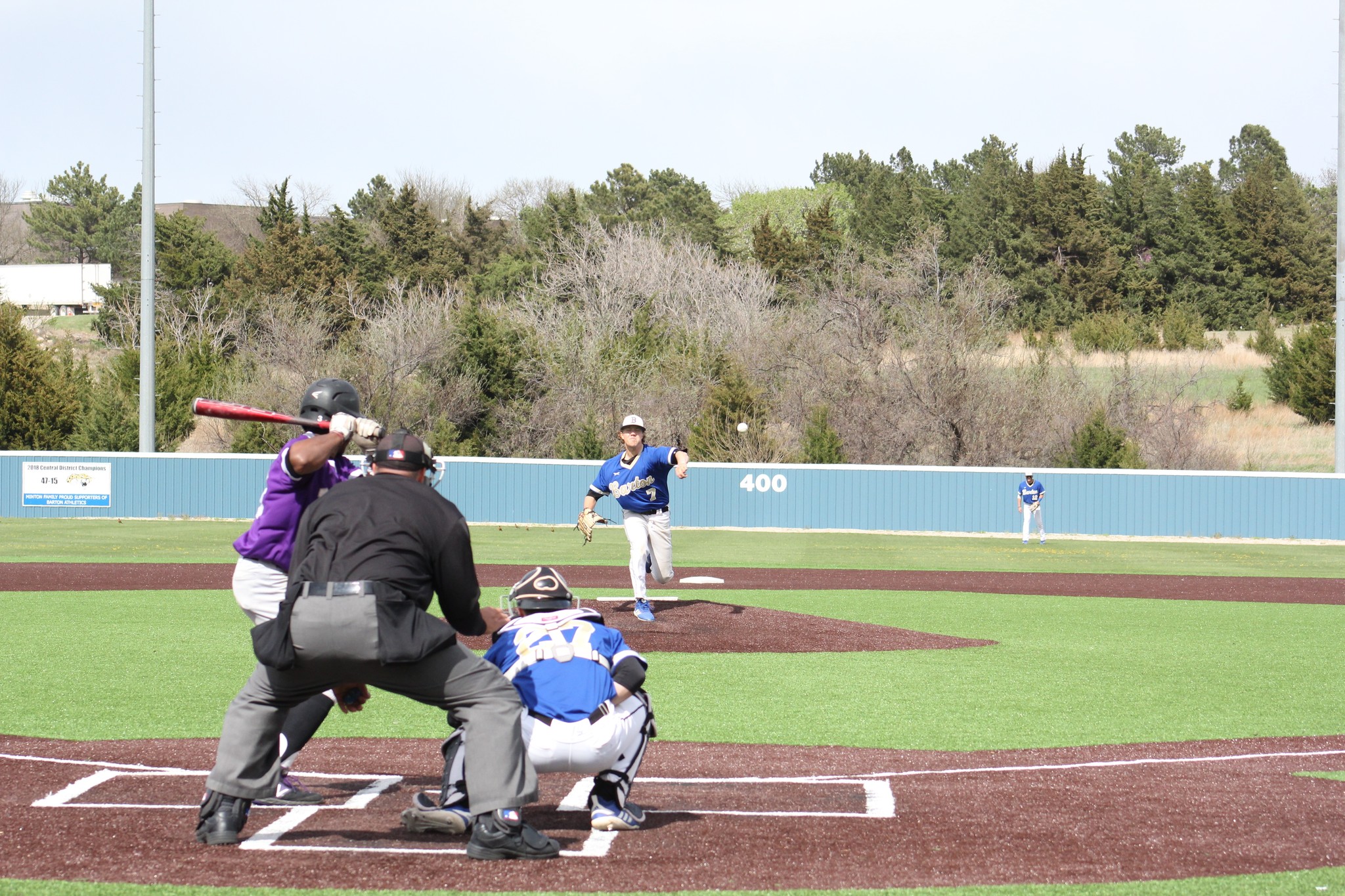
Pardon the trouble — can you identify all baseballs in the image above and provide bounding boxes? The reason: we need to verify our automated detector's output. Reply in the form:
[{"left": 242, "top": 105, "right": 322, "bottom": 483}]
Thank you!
[{"left": 737, "top": 423, "right": 748, "bottom": 433}]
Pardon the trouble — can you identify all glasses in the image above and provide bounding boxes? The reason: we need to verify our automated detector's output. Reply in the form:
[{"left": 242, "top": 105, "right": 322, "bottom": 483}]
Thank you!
[{"left": 1027, "top": 476, "right": 1032, "bottom": 478}]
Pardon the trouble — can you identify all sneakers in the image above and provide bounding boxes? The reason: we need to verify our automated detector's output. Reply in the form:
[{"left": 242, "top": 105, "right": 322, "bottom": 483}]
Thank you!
[
  {"left": 1022, "top": 541, "right": 1027, "bottom": 544},
  {"left": 590, "top": 795, "right": 641, "bottom": 830},
  {"left": 645, "top": 553, "right": 652, "bottom": 573},
  {"left": 400, "top": 807, "right": 474, "bottom": 835},
  {"left": 1040, "top": 541, "right": 1045, "bottom": 544},
  {"left": 633, "top": 598, "right": 655, "bottom": 621},
  {"left": 255, "top": 775, "right": 324, "bottom": 804}
]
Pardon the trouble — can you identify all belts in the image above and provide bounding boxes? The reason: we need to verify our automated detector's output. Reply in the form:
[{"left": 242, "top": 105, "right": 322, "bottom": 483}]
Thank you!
[
  {"left": 298, "top": 580, "right": 377, "bottom": 597},
  {"left": 641, "top": 506, "right": 669, "bottom": 515},
  {"left": 527, "top": 701, "right": 609, "bottom": 726}
]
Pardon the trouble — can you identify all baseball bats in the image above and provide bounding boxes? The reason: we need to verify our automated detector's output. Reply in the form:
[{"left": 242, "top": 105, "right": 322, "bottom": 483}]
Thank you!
[{"left": 192, "top": 396, "right": 387, "bottom": 440}]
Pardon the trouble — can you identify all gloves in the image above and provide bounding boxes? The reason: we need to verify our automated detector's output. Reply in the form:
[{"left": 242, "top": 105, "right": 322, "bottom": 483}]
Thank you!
[
  {"left": 351, "top": 417, "right": 382, "bottom": 452},
  {"left": 330, "top": 412, "right": 357, "bottom": 440}
]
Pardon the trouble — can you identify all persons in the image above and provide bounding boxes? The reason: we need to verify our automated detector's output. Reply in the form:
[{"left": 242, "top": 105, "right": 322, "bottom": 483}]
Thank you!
[
  {"left": 196, "top": 430, "right": 561, "bottom": 862},
  {"left": 229, "top": 375, "right": 385, "bottom": 809},
  {"left": 398, "top": 564, "right": 657, "bottom": 835},
  {"left": 1016, "top": 472, "right": 1046, "bottom": 546},
  {"left": 575, "top": 413, "right": 690, "bottom": 623}
]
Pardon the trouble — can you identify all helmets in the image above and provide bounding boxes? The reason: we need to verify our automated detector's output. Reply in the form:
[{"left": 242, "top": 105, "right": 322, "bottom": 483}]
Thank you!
[
  {"left": 299, "top": 377, "right": 364, "bottom": 434},
  {"left": 509, "top": 566, "right": 572, "bottom": 610}
]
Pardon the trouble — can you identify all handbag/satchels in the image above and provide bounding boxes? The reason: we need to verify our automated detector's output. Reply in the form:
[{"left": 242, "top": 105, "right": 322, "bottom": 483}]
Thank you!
[
  {"left": 375, "top": 582, "right": 457, "bottom": 665},
  {"left": 251, "top": 599, "right": 299, "bottom": 671}
]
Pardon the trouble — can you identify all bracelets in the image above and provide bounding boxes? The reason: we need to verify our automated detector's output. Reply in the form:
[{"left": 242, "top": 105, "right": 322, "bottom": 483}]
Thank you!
[{"left": 1038, "top": 498, "right": 1042, "bottom": 502}]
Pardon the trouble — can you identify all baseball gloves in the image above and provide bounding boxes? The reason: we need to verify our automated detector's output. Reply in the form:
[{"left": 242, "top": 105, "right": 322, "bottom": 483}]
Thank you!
[
  {"left": 573, "top": 507, "right": 617, "bottom": 547},
  {"left": 1029, "top": 501, "right": 1040, "bottom": 512}
]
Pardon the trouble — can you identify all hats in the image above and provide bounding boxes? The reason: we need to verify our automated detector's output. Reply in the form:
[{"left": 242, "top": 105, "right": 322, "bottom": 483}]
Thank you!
[
  {"left": 621, "top": 415, "right": 647, "bottom": 431},
  {"left": 1026, "top": 472, "right": 1033, "bottom": 476},
  {"left": 377, "top": 433, "right": 434, "bottom": 469}
]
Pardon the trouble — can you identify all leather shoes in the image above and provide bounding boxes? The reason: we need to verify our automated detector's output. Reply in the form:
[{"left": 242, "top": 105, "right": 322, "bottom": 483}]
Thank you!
[
  {"left": 467, "top": 823, "right": 559, "bottom": 860},
  {"left": 195, "top": 802, "right": 243, "bottom": 845}
]
